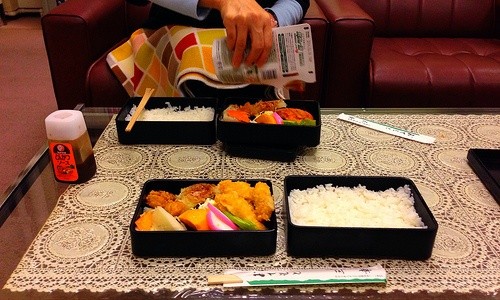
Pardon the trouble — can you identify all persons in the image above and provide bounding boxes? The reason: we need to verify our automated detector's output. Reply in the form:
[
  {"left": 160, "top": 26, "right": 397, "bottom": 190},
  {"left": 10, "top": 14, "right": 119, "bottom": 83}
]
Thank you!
[{"left": 146, "top": 0, "right": 310, "bottom": 100}]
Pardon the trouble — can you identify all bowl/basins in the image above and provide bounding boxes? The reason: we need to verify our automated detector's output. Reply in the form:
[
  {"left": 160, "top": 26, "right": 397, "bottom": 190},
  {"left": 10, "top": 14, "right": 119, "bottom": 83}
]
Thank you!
[
  {"left": 283, "top": 173, "right": 438, "bottom": 261},
  {"left": 130, "top": 178, "right": 277, "bottom": 256},
  {"left": 116, "top": 97, "right": 322, "bottom": 154}
]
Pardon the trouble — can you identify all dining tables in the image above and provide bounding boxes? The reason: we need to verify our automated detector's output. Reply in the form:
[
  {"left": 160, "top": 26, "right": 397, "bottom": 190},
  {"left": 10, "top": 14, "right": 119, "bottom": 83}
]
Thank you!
[{"left": 0, "top": 101, "right": 500, "bottom": 300}]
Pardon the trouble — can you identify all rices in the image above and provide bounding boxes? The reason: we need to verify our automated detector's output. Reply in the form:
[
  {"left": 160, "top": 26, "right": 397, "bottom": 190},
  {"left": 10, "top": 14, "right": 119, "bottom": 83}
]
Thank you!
[
  {"left": 288, "top": 183, "right": 430, "bottom": 229},
  {"left": 125, "top": 107, "right": 214, "bottom": 123}
]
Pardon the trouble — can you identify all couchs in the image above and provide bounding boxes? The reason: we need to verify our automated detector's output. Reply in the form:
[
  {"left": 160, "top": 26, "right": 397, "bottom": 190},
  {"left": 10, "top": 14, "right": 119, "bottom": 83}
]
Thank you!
[
  {"left": 320, "top": 0, "right": 500, "bottom": 109},
  {"left": 42, "top": 0, "right": 331, "bottom": 113}
]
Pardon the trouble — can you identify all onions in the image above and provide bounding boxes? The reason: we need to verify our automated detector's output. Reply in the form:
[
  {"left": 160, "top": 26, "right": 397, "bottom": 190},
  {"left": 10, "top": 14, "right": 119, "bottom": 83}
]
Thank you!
[{"left": 205, "top": 203, "right": 239, "bottom": 231}]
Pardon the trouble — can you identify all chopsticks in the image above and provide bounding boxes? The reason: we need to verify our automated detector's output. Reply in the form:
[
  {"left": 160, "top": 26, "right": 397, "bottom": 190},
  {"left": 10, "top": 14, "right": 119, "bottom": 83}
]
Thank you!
[{"left": 125, "top": 86, "right": 154, "bottom": 130}]
